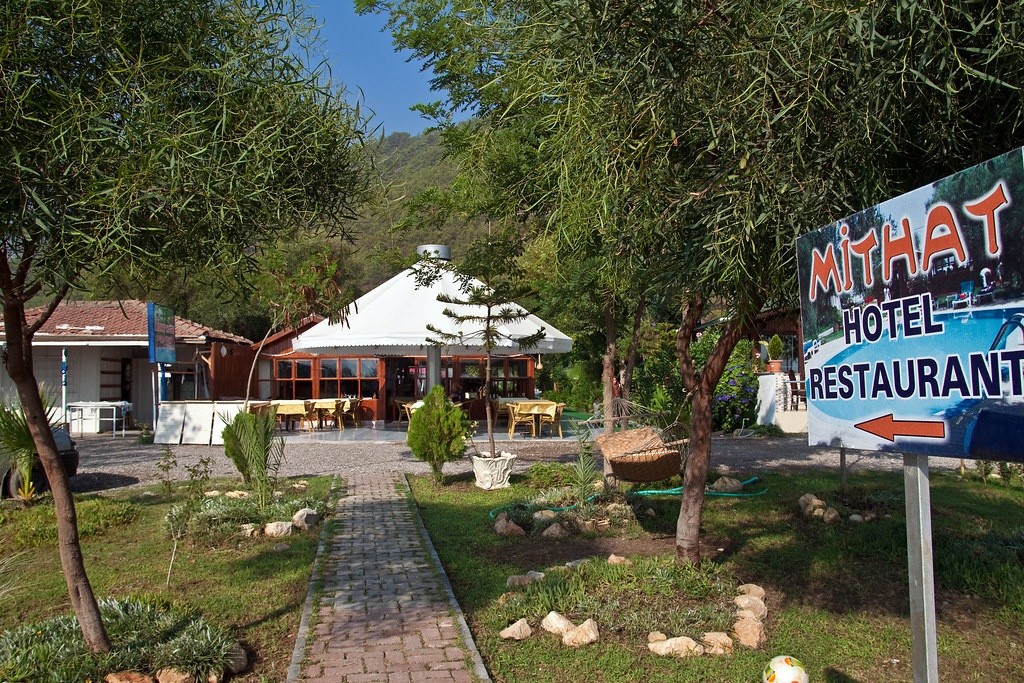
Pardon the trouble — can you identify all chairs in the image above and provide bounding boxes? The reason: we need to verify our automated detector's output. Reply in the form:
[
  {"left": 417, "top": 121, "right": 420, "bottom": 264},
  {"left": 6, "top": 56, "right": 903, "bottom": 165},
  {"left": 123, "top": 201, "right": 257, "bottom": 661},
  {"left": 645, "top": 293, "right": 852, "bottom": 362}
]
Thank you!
[
  {"left": 251, "top": 403, "right": 269, "bottom": 415},
  {"left": 492, "top": 400, "right": 508, "bottom": 427},
  {"left": 788, "top": 368, "right": 807, "bottom": 411},
  {"left": 394, "top": 399, "right": 413, "bottom": 423},
  {"left": 344, "top": 399, "right": 364, "bottom": 428},
  {"left": 506, "top": 402, "right": 536, "bottom": 439},
  {"left": 538, "top": 403, "right": 566, "bottom": 439},
  {"left": 298, "top": 402, "right": 317, "bottom": 433},
  {"left": 320, "top": 400, "right": 347, "bottom": 431},
  {"left": 402, "top": 404, "right": 414, "bottom": 440},
  {"left": 454, "top": 403, "right": 463, "bottom": 410},
  {"left": 461, "top": 399, "right": 475, "bottom": 420},
  {"left": 268, "top": 403, "right": 280, "bottom": 432}
]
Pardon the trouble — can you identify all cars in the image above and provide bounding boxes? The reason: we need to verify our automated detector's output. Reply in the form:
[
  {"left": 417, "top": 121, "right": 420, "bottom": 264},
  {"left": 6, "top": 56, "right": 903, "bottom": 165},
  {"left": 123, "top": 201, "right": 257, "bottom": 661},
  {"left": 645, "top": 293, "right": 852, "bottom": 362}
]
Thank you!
[{"left": 0, "top": 426, "right": 79, "bottom": 500}]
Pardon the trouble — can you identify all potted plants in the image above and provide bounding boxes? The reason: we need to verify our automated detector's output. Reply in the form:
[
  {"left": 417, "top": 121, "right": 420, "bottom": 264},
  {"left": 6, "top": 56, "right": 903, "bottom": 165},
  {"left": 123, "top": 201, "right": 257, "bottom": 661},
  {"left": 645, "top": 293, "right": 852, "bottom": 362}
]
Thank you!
[
  {"left": 751, "top": 358, "right": 761, "bottom": 373},
  {"left": 767, "top": 334, "right": 784, "bottom": 372},
  {"left": 766, "top": 356, "right": 771, "bottom": 372}
]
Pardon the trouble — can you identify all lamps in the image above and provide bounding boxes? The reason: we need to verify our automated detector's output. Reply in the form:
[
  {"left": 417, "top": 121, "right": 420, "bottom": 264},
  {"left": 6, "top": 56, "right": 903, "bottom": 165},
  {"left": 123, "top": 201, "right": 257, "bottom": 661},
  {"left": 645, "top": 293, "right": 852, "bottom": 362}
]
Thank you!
[{"left": 537, "top": 353, "right": 543, "bottom": 369}]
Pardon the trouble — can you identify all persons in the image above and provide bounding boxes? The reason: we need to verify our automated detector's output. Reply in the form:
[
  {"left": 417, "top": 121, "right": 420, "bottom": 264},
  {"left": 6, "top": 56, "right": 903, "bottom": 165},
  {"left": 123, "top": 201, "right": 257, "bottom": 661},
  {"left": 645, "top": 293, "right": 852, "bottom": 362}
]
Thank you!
[
  {"left": 449, "top": 383, "right": 462, "bottom": 403},
  {"left": 906, "top": 282, "right": 914, "bottom": 295},
  {"left": 884, "top": 284, "right": 891, "bottom": 301}
]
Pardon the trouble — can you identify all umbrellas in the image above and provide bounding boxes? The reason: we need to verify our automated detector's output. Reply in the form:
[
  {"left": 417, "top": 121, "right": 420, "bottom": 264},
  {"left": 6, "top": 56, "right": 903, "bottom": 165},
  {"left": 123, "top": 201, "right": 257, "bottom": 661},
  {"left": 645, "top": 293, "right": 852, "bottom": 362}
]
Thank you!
[{"left": 292, "top": 244, "right": 574, "bottom": 392}]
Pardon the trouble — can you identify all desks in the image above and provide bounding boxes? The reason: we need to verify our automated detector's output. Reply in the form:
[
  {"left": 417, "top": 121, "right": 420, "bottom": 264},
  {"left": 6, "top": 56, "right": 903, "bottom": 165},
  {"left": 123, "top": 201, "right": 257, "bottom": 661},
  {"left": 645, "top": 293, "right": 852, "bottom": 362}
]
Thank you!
[
  {"left": 497, "top": 397, "right": 528, "bottom": 427},
  {"left": 512, "top": 400, "right": 556, "bottom": 436},
  {"left": 271, "top": 400, "right": 310, "bottom": 433},
  {"left": 309, "top": 399, "right": 342, "bottom": 430},
  {"left": 410, "top": 403, "right": 425, "bottom": 413},
  {"left": 66, "top": 400, "right": 132, "bottom": 439},
  {"left": 341, "top": 398, "right": 357, "bottom": 425}
]
